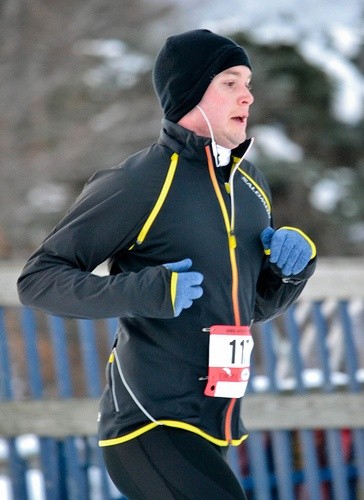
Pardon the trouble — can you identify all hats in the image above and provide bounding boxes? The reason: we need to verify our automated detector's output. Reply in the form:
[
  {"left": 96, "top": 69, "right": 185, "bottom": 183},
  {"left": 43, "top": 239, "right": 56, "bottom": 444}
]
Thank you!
[{"left": 153, "top": 29, "right": 254, "bottom": 126}]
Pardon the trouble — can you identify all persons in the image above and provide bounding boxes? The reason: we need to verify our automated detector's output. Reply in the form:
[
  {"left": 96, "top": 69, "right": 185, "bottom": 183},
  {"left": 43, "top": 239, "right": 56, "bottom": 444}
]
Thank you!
[{"left": 17, "top": 28, "right": 317, "bottom": 500}]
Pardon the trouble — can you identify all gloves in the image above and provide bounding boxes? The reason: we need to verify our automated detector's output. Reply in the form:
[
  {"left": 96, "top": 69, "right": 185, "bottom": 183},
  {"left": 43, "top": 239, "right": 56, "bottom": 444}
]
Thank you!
[
  {"left": 261, "top": 224, "right": 320, "bottom": 277},
  {"left": 159, "top": 258, "right": 204, "bottom": 319}
]
